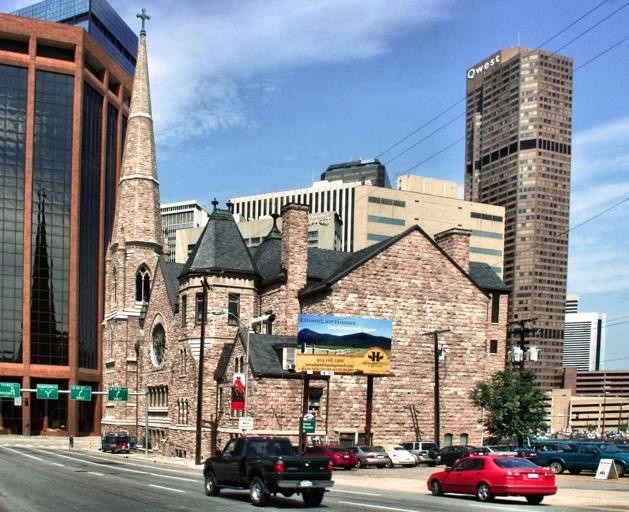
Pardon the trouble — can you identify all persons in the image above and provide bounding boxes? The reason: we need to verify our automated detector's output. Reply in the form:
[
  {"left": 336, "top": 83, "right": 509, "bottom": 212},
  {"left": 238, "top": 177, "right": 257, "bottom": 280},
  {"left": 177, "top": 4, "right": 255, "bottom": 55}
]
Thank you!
[{"left": 69, "top": 435, "right": 74, "bottom": 448}]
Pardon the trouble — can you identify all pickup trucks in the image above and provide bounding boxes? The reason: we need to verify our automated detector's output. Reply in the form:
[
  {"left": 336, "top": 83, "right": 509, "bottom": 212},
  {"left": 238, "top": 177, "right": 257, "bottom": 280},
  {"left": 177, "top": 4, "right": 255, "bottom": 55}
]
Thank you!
[
  {"left": 535, "top": 440, "right": 629, "bottom": 477},
  {"left": 202, "top": 437, "right": 334, "bottom": 507}
]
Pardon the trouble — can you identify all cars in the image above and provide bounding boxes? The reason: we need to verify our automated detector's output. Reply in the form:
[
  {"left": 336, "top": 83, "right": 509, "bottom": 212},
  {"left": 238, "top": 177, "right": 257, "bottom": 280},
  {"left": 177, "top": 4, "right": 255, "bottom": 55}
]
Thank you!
[
  {"left": 425, "top": 454, "right": 560, "bottom": 507},
  {"left": 299, "top": 439, "right": 518, "bottom": 468}
]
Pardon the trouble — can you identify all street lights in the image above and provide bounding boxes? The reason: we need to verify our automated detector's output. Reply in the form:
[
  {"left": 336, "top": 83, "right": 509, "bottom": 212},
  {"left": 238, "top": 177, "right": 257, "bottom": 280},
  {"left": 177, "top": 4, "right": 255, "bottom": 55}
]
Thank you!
[{"left": 209, "top": 308, "right": 250, "bottom": 432}]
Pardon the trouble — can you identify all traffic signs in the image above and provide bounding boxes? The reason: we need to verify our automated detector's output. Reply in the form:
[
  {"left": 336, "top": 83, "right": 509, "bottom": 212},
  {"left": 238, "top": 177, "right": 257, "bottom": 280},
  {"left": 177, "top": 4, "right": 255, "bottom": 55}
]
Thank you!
[
  {"left": 36, "top": 383, "right": 59, "bottom": 401},
  {"left": 0, "top": 381, "right": 20, "bottom": 400},
  {"left": 70, "top": 385, "right": 92, "bottom": 402},
  {"left": 107, "top": 386, "right": 128, "bottom": 403}
]
